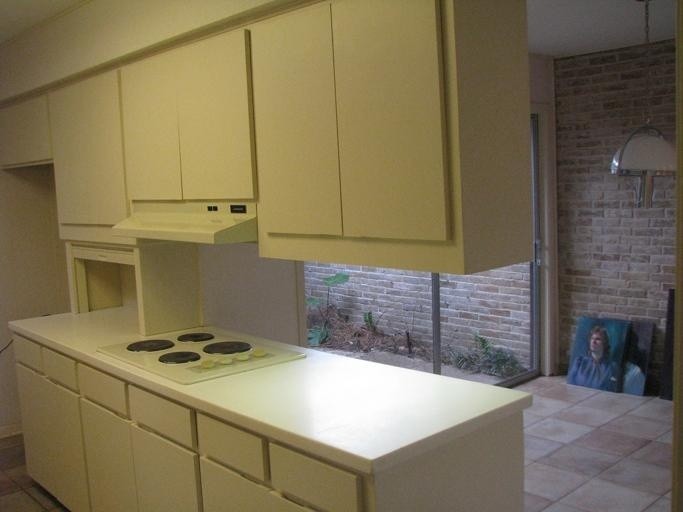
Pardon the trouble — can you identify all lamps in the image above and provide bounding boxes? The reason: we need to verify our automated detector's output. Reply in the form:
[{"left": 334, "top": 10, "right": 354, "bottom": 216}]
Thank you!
[{"left": 609, "top": 1, "right": 678, "bottom": 208}]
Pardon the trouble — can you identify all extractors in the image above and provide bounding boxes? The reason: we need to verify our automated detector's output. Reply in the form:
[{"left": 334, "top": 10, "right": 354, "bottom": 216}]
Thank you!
[{"left": 111, "top": 204, "right": 257, "bottom": 246}]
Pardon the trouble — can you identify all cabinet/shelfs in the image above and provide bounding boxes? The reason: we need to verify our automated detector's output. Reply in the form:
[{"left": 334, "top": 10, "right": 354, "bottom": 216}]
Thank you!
[
  {"left": 251, "top": 1, "right": 535, "bottom": 273},
  {"left": 12, "top": 333, "right": 86, "bottom": 512},
  {"left": 0, "top": 14, "right": 53, "bottom": 172},
  {"left": 127, "top": 381, "right": 201, "bottom": 510},
  {"left": 118, "top": 2, "right": 256, "bottom": 216},
  {"left": 75, "top": 358, "right": 137, "bottom": 511},
  {"left": 46, "top": 0, "right": 131, "bottom": 243},
  {"left": 194, "top": 408, "right": 363, "bottom": 509}
]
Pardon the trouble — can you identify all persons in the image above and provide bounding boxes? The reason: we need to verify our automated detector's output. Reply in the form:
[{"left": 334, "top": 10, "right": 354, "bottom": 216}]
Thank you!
[{"left": 567, "top": 324, "right": 619, "bottom": 391}]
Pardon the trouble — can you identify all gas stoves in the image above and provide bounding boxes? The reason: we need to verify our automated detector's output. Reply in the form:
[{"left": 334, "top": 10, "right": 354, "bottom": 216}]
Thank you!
[{"left": 98, "top": 324, "right": 305, "bottom": 386}]
